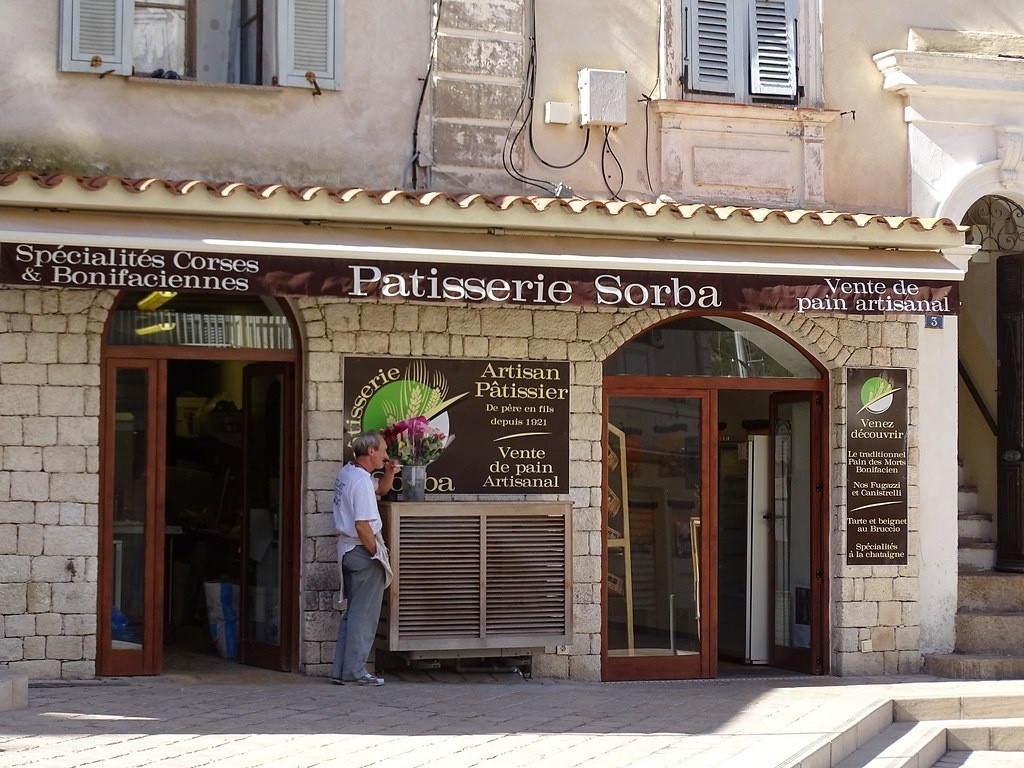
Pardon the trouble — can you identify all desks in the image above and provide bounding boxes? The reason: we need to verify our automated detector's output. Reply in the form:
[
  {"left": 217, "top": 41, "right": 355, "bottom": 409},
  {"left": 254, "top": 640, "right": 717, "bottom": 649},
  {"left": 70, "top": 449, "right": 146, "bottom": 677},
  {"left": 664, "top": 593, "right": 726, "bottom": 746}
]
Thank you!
[
  {"left": 114, "top": 524, "right": 183, "bottom": 642},
  {"left": 113, "top": 539, "right": 123, "bottom": 611}
]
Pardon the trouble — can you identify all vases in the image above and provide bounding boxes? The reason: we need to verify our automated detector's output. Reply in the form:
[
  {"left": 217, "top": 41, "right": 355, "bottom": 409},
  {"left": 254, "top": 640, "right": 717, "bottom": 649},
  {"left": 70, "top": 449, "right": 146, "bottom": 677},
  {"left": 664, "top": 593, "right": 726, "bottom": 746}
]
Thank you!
[{"left": 401, "top": 463, "right": 427, "bottom": 504}]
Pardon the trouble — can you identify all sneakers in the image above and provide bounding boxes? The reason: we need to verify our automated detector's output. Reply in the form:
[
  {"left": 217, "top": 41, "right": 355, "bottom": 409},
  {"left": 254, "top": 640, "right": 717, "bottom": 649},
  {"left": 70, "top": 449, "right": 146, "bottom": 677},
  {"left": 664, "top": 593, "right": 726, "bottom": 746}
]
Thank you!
[
  {"left": 341, "top": 673, "right": 383, "bottom": 686},
  {"left": 331, "top": 675, "right": 342, "bottom": 683}
]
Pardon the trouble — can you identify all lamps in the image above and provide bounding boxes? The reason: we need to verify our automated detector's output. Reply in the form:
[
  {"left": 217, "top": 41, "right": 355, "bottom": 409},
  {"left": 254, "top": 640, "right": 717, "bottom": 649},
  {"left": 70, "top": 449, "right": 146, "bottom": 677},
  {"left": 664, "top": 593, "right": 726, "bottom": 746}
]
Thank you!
[
  {"left": 134, "top": 322, "right": 176, "bottom": 337},
  {"left": 137, "top": 290, "right": 178, "bottom": 312}
]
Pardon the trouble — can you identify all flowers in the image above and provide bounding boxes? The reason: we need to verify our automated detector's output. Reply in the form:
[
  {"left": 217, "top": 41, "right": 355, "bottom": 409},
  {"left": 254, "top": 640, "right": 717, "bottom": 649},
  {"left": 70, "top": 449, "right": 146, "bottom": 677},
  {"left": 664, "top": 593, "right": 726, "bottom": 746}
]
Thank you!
[{"left": 377, "top": 419, "right": 448, "bottom": 467}]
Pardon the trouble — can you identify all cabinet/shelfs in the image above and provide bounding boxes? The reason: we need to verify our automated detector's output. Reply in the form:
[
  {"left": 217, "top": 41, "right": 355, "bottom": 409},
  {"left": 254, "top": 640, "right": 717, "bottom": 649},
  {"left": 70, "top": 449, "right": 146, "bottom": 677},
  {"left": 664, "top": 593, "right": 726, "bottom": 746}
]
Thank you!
[
  {"left": 115, "top": 411, "right": 137, "bottom": 478},
  {"left": 376, "top": 500, "right": 577, "bottom": 661},
  {"left": 607, "top": 423, "right": 635, "bottom": 656}
]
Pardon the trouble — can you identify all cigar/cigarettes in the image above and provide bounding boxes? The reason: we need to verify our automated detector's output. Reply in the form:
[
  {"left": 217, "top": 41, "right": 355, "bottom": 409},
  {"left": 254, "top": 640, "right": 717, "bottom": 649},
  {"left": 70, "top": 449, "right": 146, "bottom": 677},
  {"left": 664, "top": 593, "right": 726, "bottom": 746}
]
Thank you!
[{"left": 391, "top": 465, "right": 404, "bottom": 468}]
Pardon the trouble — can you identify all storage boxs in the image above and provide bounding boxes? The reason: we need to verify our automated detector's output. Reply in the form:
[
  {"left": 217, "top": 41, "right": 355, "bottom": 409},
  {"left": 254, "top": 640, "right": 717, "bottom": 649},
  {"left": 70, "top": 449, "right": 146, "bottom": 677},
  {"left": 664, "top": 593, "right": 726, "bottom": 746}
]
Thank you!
[
  {"left": 256, "top": 623, "right": 278, "bottom": 643},
  {"left": 175, "top": 397, "right": 210, "bottom": 439},
  {"left": 166, "top": 466, "right": 214, "bottom": 525},
  {"left": 248, "top": 586, "right": 273, "bottom": 623}
]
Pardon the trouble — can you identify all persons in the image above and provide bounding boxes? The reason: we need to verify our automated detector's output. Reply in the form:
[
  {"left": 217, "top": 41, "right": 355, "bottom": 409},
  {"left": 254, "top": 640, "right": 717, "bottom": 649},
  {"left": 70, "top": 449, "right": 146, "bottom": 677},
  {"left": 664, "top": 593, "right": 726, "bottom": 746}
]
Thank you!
[{"left": 332, "top": 428, "right": 401, "bottom": 686}]
